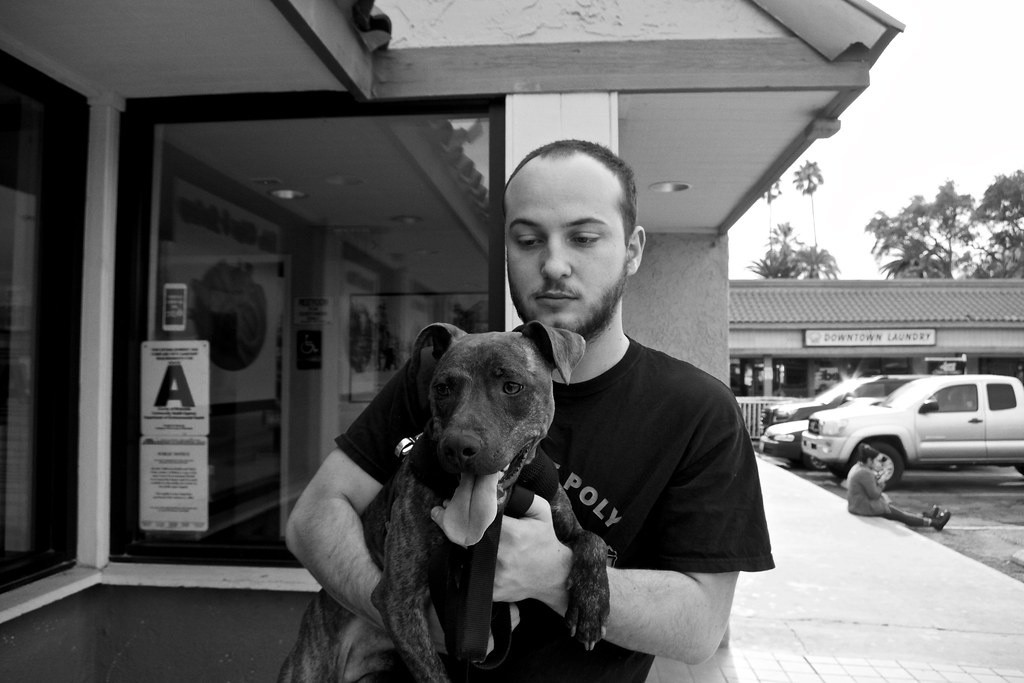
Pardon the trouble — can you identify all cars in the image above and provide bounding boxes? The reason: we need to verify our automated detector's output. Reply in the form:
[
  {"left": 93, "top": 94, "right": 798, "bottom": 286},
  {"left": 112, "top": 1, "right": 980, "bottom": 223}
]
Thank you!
[{"left": 759, "top": 372, "right": 932, "bottom": 472}]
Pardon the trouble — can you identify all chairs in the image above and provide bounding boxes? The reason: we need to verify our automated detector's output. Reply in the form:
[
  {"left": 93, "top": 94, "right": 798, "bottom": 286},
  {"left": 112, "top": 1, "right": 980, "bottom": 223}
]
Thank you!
[{"left": 948, "top": 393, "right": 966, "bottom": 410}]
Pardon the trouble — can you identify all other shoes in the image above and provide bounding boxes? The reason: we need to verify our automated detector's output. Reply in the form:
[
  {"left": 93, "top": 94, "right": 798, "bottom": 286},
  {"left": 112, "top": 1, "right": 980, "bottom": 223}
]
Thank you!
[
  {"left": 930, "top": 509, "right": 950, "bottom": 531},
  {"left": 922, "top": 505, "right": 940, "bottom": 519}
]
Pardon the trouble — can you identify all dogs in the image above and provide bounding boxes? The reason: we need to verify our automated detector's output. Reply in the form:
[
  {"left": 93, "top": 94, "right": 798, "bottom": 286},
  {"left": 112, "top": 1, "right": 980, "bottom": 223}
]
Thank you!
[{"left": 277, "top": 319, "right": 612, "bottom": 683}]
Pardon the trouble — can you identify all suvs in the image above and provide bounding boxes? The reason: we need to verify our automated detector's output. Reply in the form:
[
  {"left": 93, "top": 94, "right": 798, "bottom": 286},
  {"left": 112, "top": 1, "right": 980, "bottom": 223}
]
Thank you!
[{"left": 801, "top": 374, "right": 1024, "bottom": 492}]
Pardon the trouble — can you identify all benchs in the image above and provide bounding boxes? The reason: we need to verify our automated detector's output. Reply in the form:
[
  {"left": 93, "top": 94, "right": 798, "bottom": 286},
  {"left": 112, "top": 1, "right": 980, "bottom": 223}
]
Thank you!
[{"left": 146, "top": 407, "right": 281, "bottom": 540}]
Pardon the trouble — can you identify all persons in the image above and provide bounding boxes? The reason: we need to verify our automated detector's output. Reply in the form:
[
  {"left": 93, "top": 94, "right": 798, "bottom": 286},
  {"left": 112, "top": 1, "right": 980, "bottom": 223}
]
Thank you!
[
  {"left": 286, "top": 139, "right": 776, "bottom": 683},
  {"left": 847, "top": 443, "right": 950, "bottom": 532}
]
